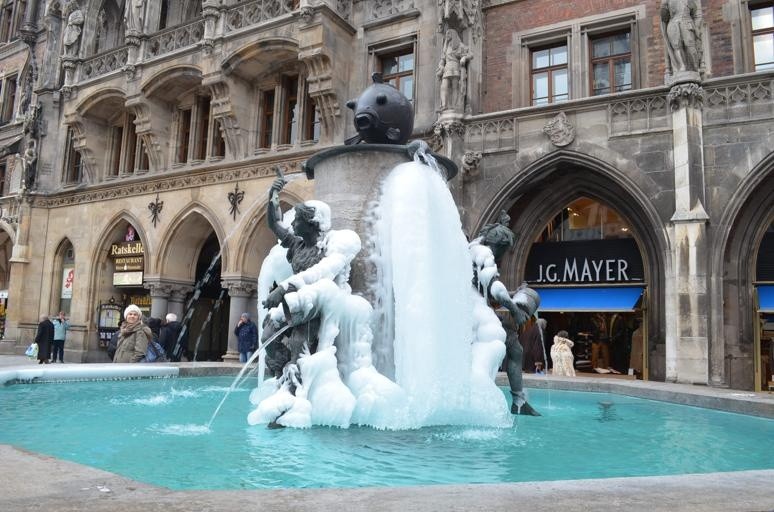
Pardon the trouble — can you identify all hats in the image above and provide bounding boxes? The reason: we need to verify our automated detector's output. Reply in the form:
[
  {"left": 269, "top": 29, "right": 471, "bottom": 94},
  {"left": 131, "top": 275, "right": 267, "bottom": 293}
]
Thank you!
[
  {"left": 124, "top": 304, "right": 143, "bottom": 319},
  {"left": 118, "top": 314, "right": 124, "bottom": 326}
]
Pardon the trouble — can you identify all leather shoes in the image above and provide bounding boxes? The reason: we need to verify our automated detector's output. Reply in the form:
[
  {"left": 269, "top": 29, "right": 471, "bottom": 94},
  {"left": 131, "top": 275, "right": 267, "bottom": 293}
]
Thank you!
[{"left": 51, "top": 359, "right": 56, "bottom": 363}]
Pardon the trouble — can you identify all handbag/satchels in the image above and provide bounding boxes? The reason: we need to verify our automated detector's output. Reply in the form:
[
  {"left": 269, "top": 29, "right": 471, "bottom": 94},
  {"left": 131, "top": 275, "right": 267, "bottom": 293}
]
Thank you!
[
  {"left": 139, "top": 340, "right": 164, "bottom": 362},
  {"left": 25, "top": 343, "right": 39, "bottom": 357}
]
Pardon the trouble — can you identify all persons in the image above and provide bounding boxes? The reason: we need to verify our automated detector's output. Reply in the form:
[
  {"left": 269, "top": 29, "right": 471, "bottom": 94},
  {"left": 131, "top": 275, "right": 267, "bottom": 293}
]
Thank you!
[
  {"left": 467, "top": 208, "right": 542, "bottom": 416},
  {"left": 51, "top": 310, "right": 70, "bottom": 363},
  {"left": 32, "top": 314, "right": 53, "bottom": 363},
  {"left": 659, "top": 0, "right": 703, "bottom": 76},
  {"left": 105, "top": 303, "right": 257, "bottom": 363},
  {"left": 260, "top": 175, "right": 362, "bottom": 430},
  {"left": 62, "top": 0, "right": 83, "bottom": 57},
  {"left": 586, "top": 311, "right": 610, "bottom": 373},
  {"left": 21, "top": 139, "right": 37, "bottom": 191},
  {"left": 523, "top": 317, "right": 548, "bottom": 374},
  {"left": 549, "top": 330, "right": 577, "bottom": 377},
  {"left": 605, "top": 312, "right": 626, "bottom": 372},
  {"left": 434, "top": 29, "right": 474, "bottom": 113}
]
